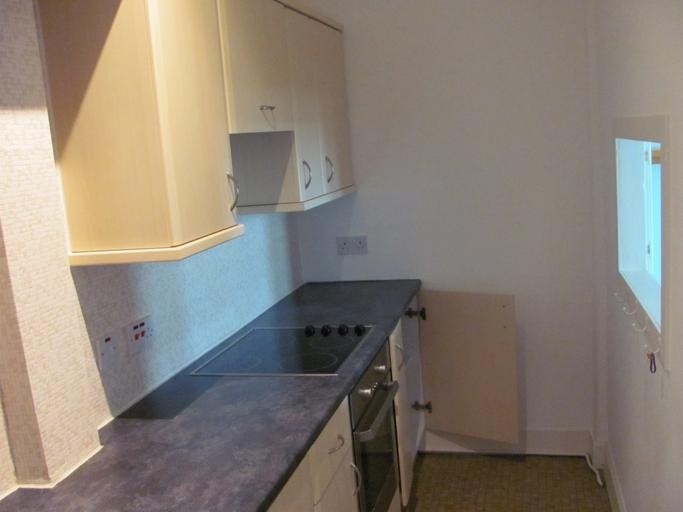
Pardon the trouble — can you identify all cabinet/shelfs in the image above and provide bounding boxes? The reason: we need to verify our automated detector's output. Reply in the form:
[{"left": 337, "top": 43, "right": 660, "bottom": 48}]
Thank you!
[
  {"left": 389, "top": 289, "right": 520, "bottom": 465},
  {"left": 217, "top": 0, "right": 295, "bottom": 134},
  {"left": 32, "top": 0, "right": 246, "bottom": 263},
  {"left": 230, "top": 0, "right": 355, "bottom": 214},
  {"left": 305, "top": 395, "right": 362, "bottom": 512}
]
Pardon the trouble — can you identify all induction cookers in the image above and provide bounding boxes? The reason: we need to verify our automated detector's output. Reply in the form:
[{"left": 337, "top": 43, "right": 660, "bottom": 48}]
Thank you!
[{"left": 193, "top": 325, "right": 375, "bottom": 379}]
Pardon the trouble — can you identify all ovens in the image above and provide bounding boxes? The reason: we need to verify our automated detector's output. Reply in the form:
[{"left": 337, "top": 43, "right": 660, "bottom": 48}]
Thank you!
[{"left": 345, "top": 338, "right": 398, "bottom": 512}]
